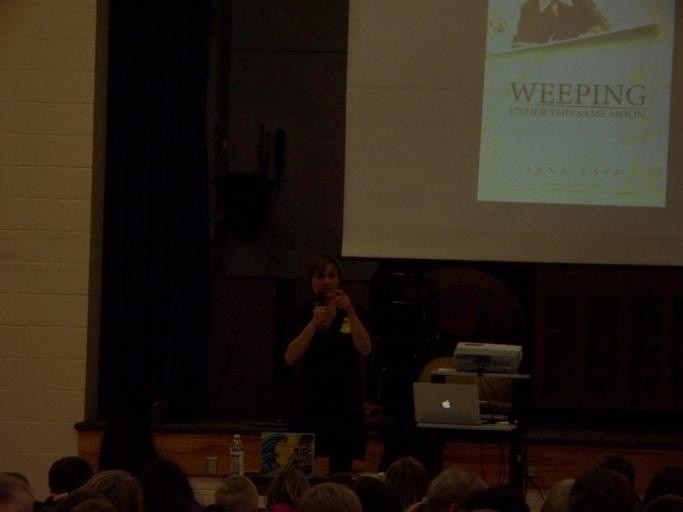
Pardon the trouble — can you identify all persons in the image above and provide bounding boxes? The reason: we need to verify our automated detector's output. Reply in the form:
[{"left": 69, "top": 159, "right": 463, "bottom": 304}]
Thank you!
[
  {"left": 275, "top": 253, "right": 373, "bottom": 477},
  {"left": 1, "top": 409, "right": 683, "bottom": 512}
]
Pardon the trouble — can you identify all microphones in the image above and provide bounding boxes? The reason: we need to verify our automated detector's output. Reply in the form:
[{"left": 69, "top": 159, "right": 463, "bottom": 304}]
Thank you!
[{"left": 317, "top": 291, "right": 327, "bottom": 329}]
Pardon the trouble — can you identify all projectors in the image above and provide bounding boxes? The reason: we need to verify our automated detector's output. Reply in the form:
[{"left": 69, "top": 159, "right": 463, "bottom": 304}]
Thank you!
[{"left": 453, "top": 341, "right": 523, "bottom": 374}]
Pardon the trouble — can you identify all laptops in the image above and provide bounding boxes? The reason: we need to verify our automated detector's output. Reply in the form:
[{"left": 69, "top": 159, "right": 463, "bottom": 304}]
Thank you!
[{"left": 412, "top": 382, "right": 488, "bottom": 425}]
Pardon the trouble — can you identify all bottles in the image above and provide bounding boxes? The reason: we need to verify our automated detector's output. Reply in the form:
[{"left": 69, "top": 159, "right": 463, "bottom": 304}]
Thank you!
[{"left": 230, "top": 434, "right": 244, "bottom": 476}]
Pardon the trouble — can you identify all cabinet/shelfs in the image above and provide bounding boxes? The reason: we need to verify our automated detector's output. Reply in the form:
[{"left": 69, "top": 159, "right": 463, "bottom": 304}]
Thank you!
[{"left": 416, "top": 370, "right": 532, "bottom": 512}]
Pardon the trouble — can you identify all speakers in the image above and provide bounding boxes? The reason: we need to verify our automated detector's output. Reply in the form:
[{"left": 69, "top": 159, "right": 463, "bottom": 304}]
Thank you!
[{"left": 222, "top": 173, "right": 269, "bottom": 232}]
